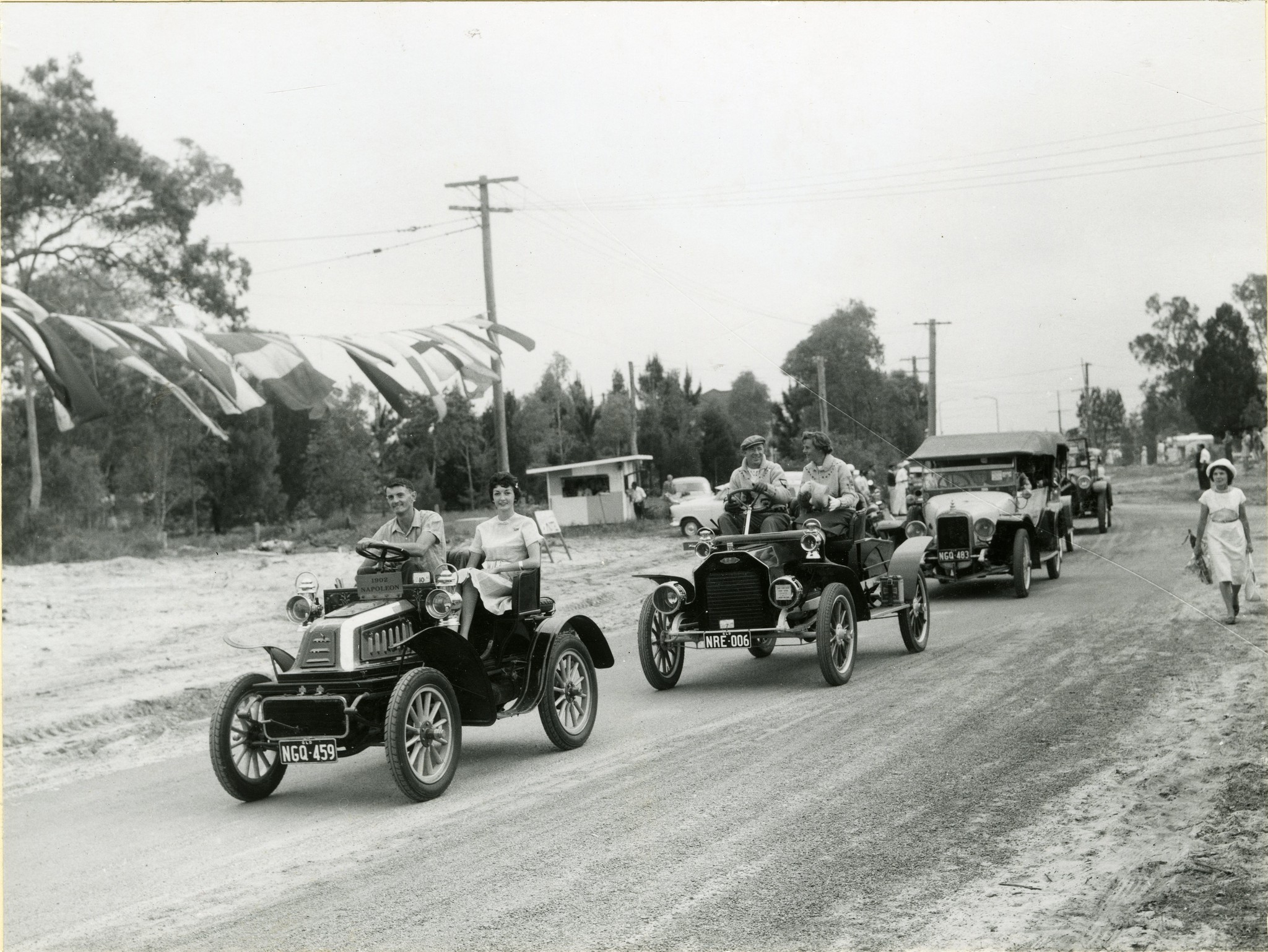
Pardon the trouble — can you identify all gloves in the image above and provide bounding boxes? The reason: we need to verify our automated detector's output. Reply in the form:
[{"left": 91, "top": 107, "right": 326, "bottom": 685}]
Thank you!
[
  {"left": 725, "top": 500, "right": 740, "bottom": 514},
  {"left": 752, "top": 482, "right": 768, "bottom": 493}
]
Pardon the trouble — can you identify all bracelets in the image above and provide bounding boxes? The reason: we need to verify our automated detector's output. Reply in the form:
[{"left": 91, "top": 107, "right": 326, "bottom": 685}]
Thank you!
[{"left": 518, "top": 560, "right": 524, "bottom": 571}]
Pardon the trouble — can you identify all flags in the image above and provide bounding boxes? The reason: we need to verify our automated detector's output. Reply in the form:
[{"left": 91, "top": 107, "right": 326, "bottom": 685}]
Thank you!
[{"left": 1, "top": 302, "right": 537, "bottom": 442}]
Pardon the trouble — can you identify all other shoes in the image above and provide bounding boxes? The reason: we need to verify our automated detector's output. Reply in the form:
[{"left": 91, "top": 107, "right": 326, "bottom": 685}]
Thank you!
[
  {"left": 1225, "top": 616, "right": 1235, "bottom": 624},
  {"left": 1233, "top": 605, "right": 1239, "bottom": 615}
]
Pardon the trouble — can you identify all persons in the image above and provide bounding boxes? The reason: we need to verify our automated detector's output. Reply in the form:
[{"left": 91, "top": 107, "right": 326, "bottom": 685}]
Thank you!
[
  {"left": 354, "top": 470, "right": 546, "bottom": 641},
  {"left": 1105, "top": 425, "right": 1264, "bottom": 624},
  {"left": 574, "top": 432, "right": 1052, "bottom": 543}
]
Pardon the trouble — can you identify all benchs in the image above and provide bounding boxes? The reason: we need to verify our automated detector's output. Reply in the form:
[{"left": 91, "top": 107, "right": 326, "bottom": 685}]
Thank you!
[{"left": 447, "top": 550, "right": 541, "bottom": 623}]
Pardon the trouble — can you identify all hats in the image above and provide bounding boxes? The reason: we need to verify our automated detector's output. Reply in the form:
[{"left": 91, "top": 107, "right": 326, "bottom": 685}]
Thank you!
[
  {"left": 1206, "top": 458, "right": 1237, "bottom": 477},
  {"left": 740, "top": 435, "right": 765, "bottom": 450}
]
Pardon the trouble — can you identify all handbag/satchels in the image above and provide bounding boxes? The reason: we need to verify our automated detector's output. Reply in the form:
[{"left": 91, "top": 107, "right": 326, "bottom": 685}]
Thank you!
[{"left": 1245, "top": 550, "right": 1261, "bottom": 602}]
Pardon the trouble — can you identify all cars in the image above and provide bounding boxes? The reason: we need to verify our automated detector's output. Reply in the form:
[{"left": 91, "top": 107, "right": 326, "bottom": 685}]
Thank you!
[
  {"left": 663, "top": 471, "right": 803, "bottom": 536},
  {"left": 208, "top": 541, "right": 614, "bottom": 819},
  {"left": 632, "top": 488, "right": 935, "bottom": 693},
  {"left": 875, "top": 429, "right": 1114, "bottom": 598}
]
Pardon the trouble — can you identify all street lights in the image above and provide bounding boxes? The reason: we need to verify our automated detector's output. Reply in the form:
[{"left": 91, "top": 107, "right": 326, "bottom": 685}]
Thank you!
[{"left": 971, "top": 396, "right": 999, "bottom": 433}]
